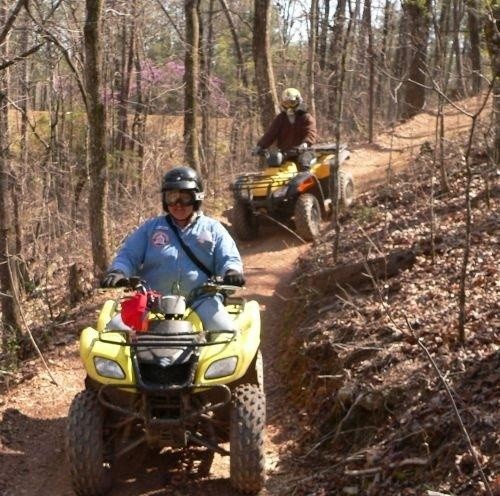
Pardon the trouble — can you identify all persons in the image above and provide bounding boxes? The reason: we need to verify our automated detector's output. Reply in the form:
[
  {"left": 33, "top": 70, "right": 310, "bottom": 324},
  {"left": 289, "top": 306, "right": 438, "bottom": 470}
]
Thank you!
[
  {"left": 99, "top": 165, "right": 247, "bottom": 342},
  {"left": 253, "top": 87, "right": 317, "bottom": 174}
]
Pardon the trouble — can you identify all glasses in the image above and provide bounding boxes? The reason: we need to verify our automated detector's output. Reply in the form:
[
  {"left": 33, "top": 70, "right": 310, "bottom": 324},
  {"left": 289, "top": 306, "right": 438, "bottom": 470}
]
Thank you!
[{"left": 163, "top": 189, "right": 195, "bottom": 207}]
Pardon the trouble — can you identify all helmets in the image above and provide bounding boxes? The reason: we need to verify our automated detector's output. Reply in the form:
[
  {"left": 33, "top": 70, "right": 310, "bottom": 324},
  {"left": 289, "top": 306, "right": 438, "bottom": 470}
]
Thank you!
[
  {"left": 281, "top": 88, "right": 302, "bottom": 113},
  {"left": 161, "top": 167, "right": 204, "bottom": 211}
]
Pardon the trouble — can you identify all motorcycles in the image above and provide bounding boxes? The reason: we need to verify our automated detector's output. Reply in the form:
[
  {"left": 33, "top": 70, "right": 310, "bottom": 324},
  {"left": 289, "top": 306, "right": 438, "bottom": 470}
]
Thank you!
[
  {"left": 225, "top": 140, "right": 355, "bottom": 241},
  {"left": 66, "top": 274, "right": 268, "bottom": 494}
]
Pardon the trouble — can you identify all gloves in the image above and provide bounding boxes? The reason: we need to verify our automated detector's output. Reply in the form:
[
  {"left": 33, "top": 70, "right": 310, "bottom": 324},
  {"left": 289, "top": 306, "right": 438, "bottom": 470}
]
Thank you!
[
  {"left": 101, "top": 271, "right": 125, "bottom": 287},
  {"left": 253, "top": 145, "right": 260, "bottom": 156},
  {"left": 298, "top": 144, "right": 308, "bottom": 153},
  {"left": 223, "top": 270, "right": 245, "bottom": 287}
]
